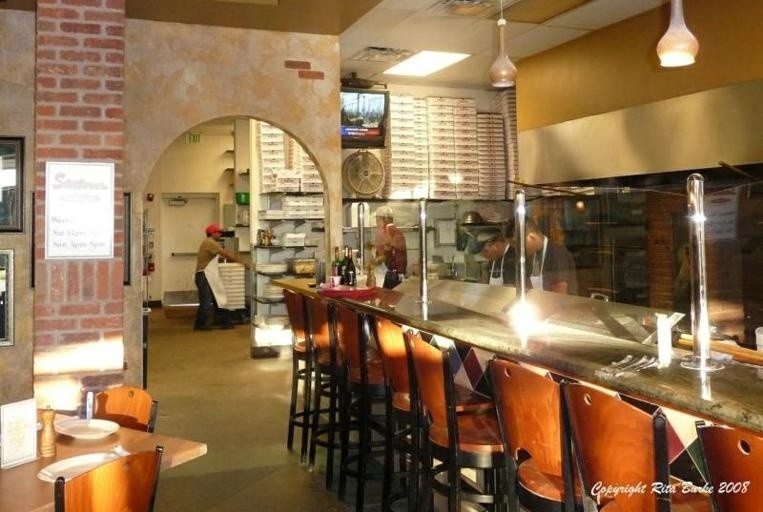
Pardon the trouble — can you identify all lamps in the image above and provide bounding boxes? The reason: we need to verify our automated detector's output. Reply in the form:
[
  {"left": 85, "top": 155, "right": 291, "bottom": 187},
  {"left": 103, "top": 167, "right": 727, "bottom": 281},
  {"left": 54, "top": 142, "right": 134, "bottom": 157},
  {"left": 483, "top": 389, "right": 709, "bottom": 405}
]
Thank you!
[
  {"left": 488, "top": 1, "right": 519, "bottom": 88},
  {"left": 654, "top": 0, "right": 699, "bottom": 69}
]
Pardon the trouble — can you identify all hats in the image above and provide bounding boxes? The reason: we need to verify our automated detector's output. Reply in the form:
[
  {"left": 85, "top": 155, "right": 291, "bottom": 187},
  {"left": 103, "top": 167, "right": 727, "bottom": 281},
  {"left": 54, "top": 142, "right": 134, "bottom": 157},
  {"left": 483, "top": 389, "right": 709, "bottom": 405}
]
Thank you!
[
  {"left": 369, "top": 205, "right": 395, "bottom": 219},
  {"left": 204, "top": 224, "right": 225, "bottom": 235},
  {"left": 468, "top": 227, "right": 503, "bottom": 256}
]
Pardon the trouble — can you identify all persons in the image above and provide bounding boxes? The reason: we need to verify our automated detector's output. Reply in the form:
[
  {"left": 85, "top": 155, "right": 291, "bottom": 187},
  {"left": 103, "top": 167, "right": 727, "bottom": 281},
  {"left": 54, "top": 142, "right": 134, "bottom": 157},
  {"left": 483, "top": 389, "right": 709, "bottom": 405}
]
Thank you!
[
  {"left": 467, "top": 228, "right": 533, "bottom": 288},
  {"left": 370, "top": 205, "right": 407, "bottom": 289},
  {"left": 505, "top": 216, "right": 578, "bottom": 294},
  {"left": 193, "top": 224, "right": 236, "bottom": 331}
]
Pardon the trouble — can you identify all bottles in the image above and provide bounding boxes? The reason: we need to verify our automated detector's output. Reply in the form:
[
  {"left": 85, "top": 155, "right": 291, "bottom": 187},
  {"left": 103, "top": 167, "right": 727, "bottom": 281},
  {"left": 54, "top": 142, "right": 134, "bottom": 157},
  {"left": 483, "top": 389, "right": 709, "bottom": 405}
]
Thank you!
[{"left": 314, "top": 244, "right": 376, "bottom": 294}]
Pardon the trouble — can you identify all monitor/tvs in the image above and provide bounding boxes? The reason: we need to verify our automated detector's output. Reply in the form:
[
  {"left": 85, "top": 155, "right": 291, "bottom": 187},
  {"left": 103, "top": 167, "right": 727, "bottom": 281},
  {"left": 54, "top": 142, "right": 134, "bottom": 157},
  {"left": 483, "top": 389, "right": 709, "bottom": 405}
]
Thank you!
[{"left": 340, "top": 88, "right": 389, "bottom": 149}]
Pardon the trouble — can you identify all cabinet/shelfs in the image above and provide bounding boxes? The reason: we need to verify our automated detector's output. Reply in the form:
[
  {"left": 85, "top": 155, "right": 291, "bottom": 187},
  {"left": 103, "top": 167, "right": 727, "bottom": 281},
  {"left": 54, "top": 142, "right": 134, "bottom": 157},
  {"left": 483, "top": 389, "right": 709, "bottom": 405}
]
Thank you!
[
  {"left": 566, "top": 237, "right": 650, "bottom": 308},
  {"left": 249, "top": 192, "right": 324, "bottom": 359}
]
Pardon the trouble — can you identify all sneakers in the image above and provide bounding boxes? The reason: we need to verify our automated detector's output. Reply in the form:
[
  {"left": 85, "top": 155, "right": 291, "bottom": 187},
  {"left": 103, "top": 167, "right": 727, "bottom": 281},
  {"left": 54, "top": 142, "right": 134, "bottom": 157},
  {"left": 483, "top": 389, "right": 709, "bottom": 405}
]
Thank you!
[{"left": 192, "top": 322, "right": 213, "bottom": 331}]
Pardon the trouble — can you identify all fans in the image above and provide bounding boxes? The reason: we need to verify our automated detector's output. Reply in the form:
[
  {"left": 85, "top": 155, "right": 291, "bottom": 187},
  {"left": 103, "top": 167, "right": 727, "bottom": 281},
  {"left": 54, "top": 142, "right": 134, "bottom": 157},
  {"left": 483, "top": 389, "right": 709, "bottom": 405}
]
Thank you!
[{"left": 342, "top": 148, "right": 387, "bottom": 199}]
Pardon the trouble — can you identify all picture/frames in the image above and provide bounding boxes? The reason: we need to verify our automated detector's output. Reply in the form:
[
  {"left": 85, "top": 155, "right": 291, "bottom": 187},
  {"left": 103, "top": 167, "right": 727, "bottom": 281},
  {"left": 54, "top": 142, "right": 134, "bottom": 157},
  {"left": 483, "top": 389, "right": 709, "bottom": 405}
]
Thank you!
[
  {"left": 0, "top": 246, "right": 16, "bottom": 349},
  {"left": 0, "top": 135, "right": 26, "bottom": 235}
]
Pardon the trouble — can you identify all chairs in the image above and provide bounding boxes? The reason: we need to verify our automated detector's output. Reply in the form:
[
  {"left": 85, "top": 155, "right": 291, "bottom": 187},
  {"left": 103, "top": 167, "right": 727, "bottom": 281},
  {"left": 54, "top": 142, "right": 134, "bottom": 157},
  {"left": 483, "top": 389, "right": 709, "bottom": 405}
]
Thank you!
[
  {"left": 280, "top": 286, "right": 762, "bottom": 512},
  {"left": 84, "top": 384, "right": 159, "bottom": 433},
  {"left": 51, "top": 443, "right": 165, "bottom": 512}
]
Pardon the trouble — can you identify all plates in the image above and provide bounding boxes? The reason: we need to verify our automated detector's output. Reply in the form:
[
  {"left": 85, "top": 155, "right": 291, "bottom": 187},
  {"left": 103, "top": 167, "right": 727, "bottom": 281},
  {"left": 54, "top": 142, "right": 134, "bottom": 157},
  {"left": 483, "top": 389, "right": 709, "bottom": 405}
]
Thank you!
[
  {"left": 36, "top": 403, "right": 122, "bottom": 486},
  {"left": 258, "top": 262, "right": 290, "bottom": 301}
]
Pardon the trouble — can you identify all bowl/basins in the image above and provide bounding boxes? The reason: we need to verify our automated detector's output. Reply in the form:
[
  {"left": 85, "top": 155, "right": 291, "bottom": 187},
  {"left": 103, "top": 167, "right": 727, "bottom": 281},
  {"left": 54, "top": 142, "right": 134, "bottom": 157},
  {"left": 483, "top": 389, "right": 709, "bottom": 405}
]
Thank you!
[{"left": 459, "top": 211, "right": 483, "bottom": 227}]
